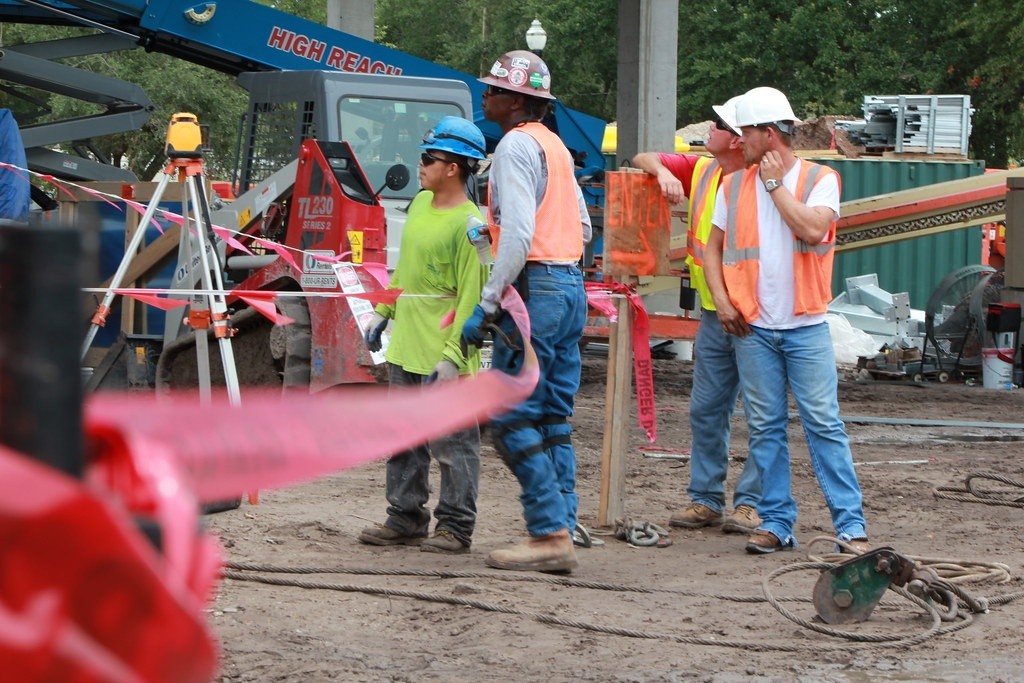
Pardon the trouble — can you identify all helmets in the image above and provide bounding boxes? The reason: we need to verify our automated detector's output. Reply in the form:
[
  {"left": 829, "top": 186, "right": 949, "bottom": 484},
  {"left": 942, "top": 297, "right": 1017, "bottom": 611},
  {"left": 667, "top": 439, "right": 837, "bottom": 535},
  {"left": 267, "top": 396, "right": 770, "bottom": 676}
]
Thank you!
[
  {"left": 713, "top": 96, "right": 741, "bottom": 137},
  {"left": 476, "top": 50, "right": 558, "bottom": 100},
  {"left": 419, "top": 115, "right": 486, "bottom": 161},
  {"left": 736, "top": 86, "right": 803, "bottom": 127}
]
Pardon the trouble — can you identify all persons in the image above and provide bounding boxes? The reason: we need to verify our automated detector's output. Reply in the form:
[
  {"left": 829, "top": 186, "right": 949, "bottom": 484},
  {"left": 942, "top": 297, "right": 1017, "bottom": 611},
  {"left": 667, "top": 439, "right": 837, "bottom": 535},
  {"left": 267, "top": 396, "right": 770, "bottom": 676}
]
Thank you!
[
  {"left": 703, "top": 90, "right": 869, "bottom": 554},
  {"left": 632, "top": 94, "right": 763, "bottom": 532},
  {"left": 460, "top": 49, "right": 590, "bottom": 571},
  {"left": 359, "top": 117, "right": 490, "bottom": 552}
]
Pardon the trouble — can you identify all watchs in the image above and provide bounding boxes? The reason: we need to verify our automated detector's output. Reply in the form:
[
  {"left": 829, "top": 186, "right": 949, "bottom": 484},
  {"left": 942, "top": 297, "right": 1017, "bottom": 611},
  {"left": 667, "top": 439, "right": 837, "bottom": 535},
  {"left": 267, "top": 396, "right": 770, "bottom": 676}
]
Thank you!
[{"left": 765, "top": 179, "right": 783, "bottom": 192}]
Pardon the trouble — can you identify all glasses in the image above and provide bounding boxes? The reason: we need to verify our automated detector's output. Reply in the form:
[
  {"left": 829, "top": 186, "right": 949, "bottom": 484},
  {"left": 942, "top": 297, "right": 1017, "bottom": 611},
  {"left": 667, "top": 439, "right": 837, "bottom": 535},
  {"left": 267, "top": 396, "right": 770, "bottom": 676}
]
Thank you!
[
  {"left": 486, "top": 85, "right": 520, "bottom": 96},
  {"left": 714, "top": 117, "right": 740, "bottom": 136},
  {"left": 420, "top": 152, "right": 464, "bottom": 168}
]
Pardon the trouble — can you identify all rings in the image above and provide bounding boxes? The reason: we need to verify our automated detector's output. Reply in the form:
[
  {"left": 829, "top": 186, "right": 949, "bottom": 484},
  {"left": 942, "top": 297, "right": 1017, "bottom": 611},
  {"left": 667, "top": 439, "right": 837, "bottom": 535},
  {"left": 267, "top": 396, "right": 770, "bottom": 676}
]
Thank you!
[{"left": 764, "top": 160, "right": 768, "bottom": 162}]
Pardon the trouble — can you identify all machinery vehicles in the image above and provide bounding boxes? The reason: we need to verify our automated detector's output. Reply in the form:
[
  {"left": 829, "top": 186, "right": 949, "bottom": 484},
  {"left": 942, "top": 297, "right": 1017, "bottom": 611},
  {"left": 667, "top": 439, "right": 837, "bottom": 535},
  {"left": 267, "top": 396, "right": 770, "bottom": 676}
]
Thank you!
[{"left": 2, "top": 0, "right": 1012, "bottom": 390}]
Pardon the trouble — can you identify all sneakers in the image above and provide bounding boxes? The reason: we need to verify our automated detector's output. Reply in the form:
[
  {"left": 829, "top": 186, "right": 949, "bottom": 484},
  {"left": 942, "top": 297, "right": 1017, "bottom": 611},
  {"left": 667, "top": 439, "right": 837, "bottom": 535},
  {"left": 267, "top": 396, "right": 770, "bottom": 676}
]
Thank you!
[
  {"left": 837, "top": 533, "right": 872, "bottom": 557},
  {"left": 417, "top": 524, "right": 474, "bottom": 554},
  {"left": 359, "top": 518, "right": 430, "bottom": 549},
  {"left": 745, "top": 516, "right": 793, "bottom": 553},
  {"left": 723, "top": 504, "right": 763, "bottom": 533},
  {"left": 670, "top": 498, "right": 724, "bottom": 526},
  {"left": 486, "top": 527, "right": 581, "bottom": 571}
]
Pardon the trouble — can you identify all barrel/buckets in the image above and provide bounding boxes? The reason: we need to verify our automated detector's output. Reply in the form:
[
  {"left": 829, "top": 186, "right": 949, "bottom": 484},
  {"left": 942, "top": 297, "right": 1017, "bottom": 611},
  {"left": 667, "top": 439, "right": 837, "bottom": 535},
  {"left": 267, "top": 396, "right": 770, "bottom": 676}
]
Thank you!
[
  {"left": 468, "top": 214, "right": 495, "bottom": 265},
  {"left": 981, "top": 347, "right": 1014, "bottom": 389}
]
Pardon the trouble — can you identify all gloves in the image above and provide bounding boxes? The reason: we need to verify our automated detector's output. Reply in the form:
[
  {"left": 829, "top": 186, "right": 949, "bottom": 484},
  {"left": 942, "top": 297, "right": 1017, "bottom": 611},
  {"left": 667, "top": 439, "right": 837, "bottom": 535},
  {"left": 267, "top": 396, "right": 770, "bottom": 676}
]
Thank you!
[
  {"left": 460, "top": 304, "right": 496, "bottom": 359},
  {"left": 426, "top": 361, "right": 459, "bottom": 405},
  {"left": 363, "top": 314, "right": 388, "bottom": 353}
]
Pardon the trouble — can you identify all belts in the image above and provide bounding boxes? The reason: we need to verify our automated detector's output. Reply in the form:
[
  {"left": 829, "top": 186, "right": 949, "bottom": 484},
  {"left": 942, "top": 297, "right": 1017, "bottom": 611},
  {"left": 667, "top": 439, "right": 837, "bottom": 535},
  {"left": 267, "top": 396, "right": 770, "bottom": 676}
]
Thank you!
[{"left": 525, "top": 260, "right": 569, "bottom": 269}]
